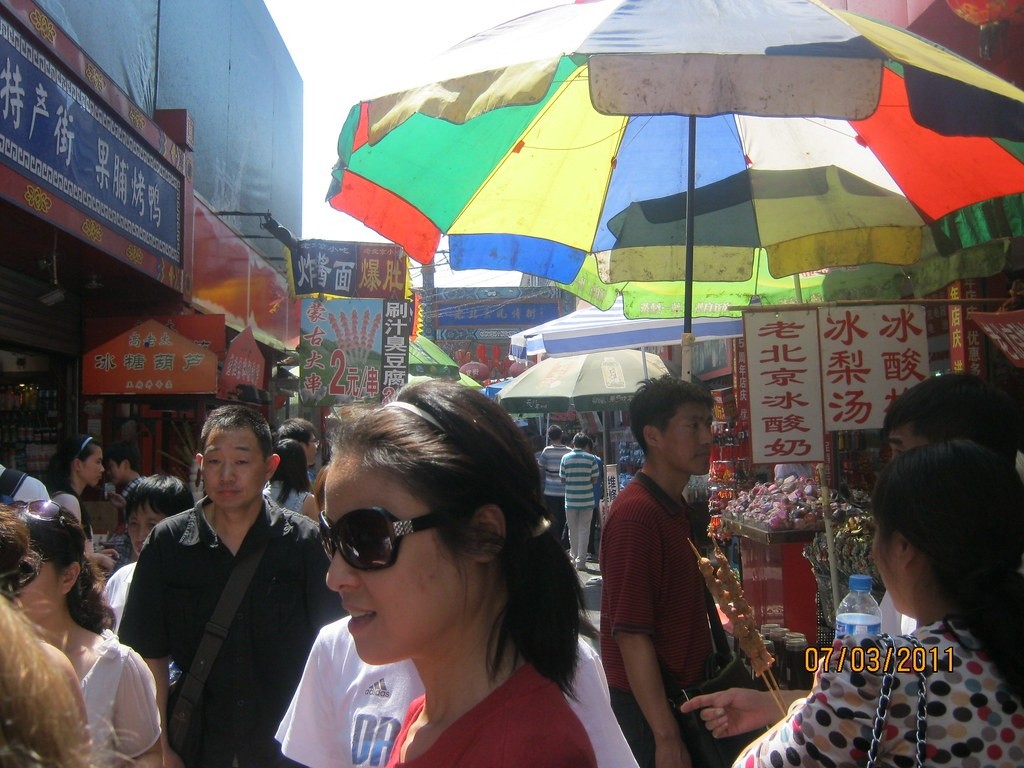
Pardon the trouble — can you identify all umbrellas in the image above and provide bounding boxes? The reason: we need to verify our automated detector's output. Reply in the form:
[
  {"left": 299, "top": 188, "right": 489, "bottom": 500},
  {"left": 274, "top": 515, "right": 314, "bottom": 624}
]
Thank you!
[{"left": 324, "top": 1, "right": 1023, "bottom": 417}]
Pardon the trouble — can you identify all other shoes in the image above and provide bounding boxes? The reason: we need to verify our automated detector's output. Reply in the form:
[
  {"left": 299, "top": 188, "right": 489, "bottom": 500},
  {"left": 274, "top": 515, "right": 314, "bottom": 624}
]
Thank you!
[{"left": 570, "top": 560, "right": 588, "bottom": 571}]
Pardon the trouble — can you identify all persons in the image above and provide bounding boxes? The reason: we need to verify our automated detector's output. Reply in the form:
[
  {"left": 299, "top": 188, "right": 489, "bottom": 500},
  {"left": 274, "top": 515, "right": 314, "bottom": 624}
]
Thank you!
[
  {"left": 325, "top": 379, "right": 600, "bottom": 767},
  {"left": 263, "top": 417, "right": 319, "bottom": 526},
  {"left": 4, "top": 500, "right": 165, "bottom": 767},
  {"left": 771, "top": 461, "right": 816, "bottom": 480},
  {"left": 519, "top": 423, "right": 604, "bottom": 571},
  {"left": 724, "top": 436, "right": 1024, "bottom": 768},
  {"left": 599, "top": 376, "right": 719, "bottom": 768},
  {"left": 680, "top": 369, "right": 1024, "bottom": 739},
  {"left": 102, "top": 476, "right": 196, "bottom": 643},
  {"left": 0, "top": 433, "right": 140, "bottom": 583},
  {"left": 120, "top": 404, "right": 348, "bottom": 768},
  {"left": 273, "top": 613, "right": 641, "bottom": 767}
]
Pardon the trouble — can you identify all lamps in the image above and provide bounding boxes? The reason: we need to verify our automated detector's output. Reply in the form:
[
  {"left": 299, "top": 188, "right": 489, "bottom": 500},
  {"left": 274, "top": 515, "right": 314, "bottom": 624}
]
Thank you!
[{"left": 216, "top": 209, "right": 307, "bottom": 261}]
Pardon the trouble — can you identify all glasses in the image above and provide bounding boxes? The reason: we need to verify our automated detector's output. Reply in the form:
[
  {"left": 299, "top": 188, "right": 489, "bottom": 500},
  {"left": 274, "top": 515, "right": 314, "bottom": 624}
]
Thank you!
[
  {"left": 318, "top": 507, "right": 447, "bottom": 573},
  {"left": 8, "top": 499, "right": 78, "bottom": 527},
  {"left": 309, "top": 439, "right": 320, "bottom": 448}
]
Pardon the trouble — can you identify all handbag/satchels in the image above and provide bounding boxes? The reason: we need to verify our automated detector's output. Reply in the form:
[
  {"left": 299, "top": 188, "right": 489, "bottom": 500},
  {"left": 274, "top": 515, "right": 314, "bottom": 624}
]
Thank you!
[
  {"left": 671, "top": 653, "right": 772, "bottom": 768},
  {"left": 165, "top": 673, "right": 206, "bottom": 768}
]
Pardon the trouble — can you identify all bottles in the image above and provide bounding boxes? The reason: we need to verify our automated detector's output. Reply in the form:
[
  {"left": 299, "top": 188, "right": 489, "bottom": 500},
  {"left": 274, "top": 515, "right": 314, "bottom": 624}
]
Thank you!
[
  {"left": 0, "top": 382, "right": 59, "bottom": 473},
  {"left": 755, "top": 623, "right": 811, "bottom": 690},
  {"left": 833, "top": 574, "right": 882, "bottom": 645}
]
